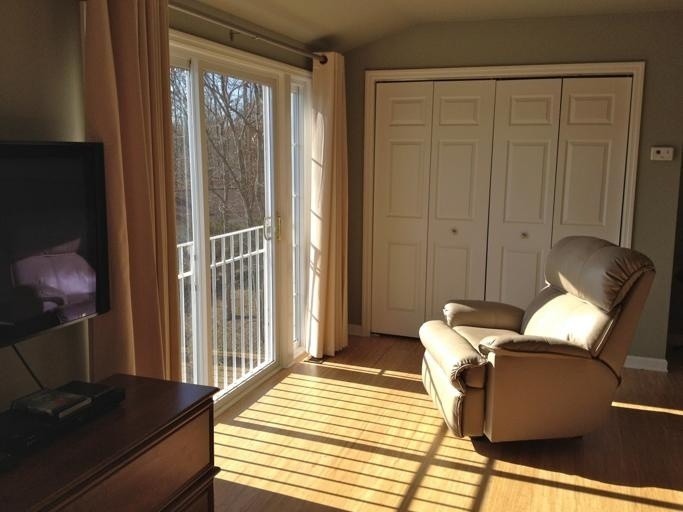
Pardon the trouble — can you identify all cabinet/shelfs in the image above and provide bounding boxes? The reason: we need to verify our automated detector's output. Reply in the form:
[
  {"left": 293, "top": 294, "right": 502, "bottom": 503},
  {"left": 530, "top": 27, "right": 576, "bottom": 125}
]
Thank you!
[{"left": 0, "top": 370, "right": 221, "bottom": 512}]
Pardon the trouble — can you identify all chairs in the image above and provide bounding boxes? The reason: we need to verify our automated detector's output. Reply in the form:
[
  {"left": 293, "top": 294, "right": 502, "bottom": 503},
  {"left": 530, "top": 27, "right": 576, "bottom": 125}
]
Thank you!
[{"left": 419, "top": 234, "right": 656, "bottom": 446}]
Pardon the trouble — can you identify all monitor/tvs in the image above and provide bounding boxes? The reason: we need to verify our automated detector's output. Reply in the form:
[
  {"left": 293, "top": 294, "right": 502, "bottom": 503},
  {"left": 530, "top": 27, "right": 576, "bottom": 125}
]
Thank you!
[{"left": 0, "top": 140, "right": 111, "bottom": 349}]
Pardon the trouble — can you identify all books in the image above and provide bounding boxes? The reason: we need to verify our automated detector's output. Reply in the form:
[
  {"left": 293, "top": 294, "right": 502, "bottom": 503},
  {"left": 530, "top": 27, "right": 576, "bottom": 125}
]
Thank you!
[
  {"left": 58, "top": 381, "right": 116, "bottom": 397},
  {"left": 11, "top": 387, "right": 91, "bottom": 419}
]
